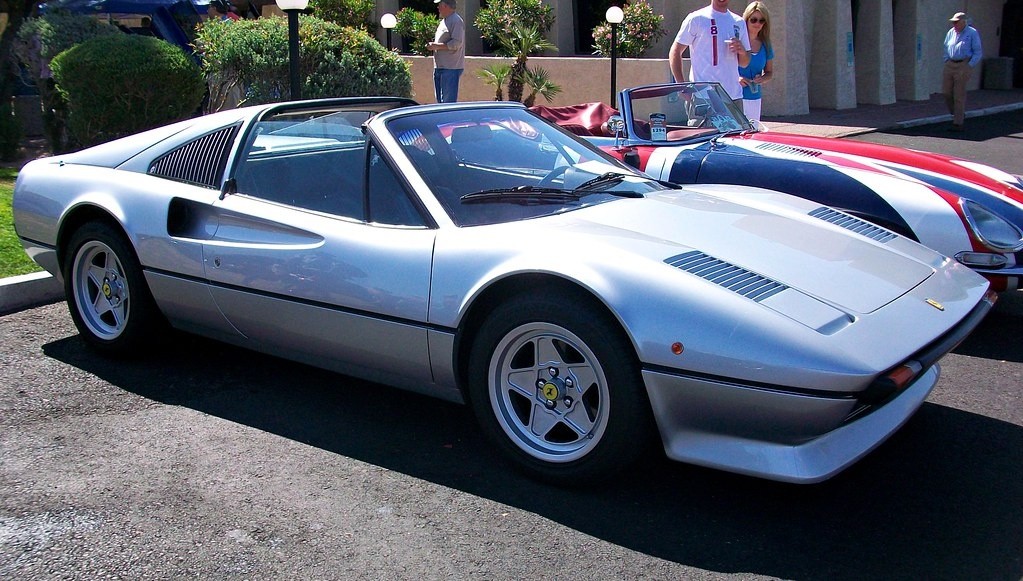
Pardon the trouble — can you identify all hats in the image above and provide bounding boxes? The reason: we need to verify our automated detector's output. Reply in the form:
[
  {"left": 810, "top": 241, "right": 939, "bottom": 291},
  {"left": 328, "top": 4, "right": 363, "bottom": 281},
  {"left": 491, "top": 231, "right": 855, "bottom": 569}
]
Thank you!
[
  {"left": 949, "top": 12, "right": 967, "bottom": 22},
  {"left": 434, "top": 0, "right": 456, "bottom": 4}
]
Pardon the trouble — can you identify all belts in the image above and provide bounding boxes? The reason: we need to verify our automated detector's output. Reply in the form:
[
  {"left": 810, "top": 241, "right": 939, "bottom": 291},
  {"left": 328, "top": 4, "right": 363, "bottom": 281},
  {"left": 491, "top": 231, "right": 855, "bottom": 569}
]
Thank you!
[{"left": 950, "top": 59, "right": 964, "bottom": 63}]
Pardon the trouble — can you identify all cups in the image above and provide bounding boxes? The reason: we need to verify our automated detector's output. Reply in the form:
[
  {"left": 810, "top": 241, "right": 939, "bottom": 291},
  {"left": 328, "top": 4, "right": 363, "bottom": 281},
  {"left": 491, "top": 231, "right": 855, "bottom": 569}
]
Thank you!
[
  {"left": 723, "top": 40, "right": 737, "bottom": 60},
  {"left": 748, "top": 80, "right": 759, "bottom": 93}
]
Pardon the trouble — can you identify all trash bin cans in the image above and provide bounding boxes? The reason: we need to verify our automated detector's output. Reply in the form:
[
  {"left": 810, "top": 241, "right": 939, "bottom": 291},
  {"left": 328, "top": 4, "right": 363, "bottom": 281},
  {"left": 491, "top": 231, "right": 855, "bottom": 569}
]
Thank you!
[{"left": 983, "top": 56, "right": 1013, "bottom": 91}]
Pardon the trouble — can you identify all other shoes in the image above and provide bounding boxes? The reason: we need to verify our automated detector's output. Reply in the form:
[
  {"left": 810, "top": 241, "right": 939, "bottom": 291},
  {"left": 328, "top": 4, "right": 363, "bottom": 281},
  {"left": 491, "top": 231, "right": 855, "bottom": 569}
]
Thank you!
[{"left": 949, "top": 124, "right": 963, "bottom": 131}]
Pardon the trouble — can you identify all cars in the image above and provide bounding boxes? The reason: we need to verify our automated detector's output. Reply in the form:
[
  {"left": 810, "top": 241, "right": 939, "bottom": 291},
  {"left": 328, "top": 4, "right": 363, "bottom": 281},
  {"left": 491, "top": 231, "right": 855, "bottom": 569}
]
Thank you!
[{"left": 38, "top": 0, "right": 206, "bottom": 73}]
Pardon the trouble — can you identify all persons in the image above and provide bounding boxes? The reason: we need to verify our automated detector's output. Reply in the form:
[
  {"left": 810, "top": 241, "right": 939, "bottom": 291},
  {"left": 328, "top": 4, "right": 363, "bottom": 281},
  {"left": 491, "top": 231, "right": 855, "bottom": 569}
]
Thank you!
[
  {"left": 424, "top": 0, "right": 465, "bottom": 103},
  {"left": 942, "top": 11, "right": 983, "bottom": 133},
  {"left": 192, "top": 0, "right": 246, "bottom": 108},
  {"left": 670, "top": 1, "right": 774, "bottom": 131}
]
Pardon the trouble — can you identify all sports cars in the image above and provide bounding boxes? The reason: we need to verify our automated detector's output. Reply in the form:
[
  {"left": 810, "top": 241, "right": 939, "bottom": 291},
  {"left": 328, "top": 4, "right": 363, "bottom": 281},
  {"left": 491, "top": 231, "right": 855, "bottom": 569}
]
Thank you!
[
  {"left": 10, "top": 95, "right": 999, "bottom": 492},
  {"left": 520, "top": 80, "right": 1023, "bottom": 306}
]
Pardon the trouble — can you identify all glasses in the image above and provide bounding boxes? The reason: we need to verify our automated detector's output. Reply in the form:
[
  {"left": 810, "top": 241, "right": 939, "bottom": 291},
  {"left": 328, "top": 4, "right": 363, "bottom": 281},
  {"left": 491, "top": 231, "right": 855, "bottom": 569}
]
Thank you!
[{"left": 749, "top": 16, "right": 766, "bottom": 24}]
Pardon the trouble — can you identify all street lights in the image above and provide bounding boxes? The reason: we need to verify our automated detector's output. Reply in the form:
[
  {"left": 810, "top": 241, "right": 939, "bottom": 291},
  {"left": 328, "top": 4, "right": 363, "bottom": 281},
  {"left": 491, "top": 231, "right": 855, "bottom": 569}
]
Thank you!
[
  {"left": 275, "top": 0, "right": 311, "bottom": 102},
  {"left": 606, "top": 6, "right": 624, "bottom": 109},
  {"left": 380, "top": 13, "right": 399, "bottom": 55}
]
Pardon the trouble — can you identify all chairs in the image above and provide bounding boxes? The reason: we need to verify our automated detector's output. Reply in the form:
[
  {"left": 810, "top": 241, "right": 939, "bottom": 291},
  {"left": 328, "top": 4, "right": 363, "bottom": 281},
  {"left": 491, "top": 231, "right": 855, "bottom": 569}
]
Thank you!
[
  {"left": 369, "top": 145, "right": 454, "bottom": 223},
  {"left": 247, "top": 154, "right": 357, "bottom": 216},
  {"left": 560, "top": 123, "right": 593, "bottom": 136},
  {"left": 601, "top": 118, "right": 648, "bottom": 137}
]
